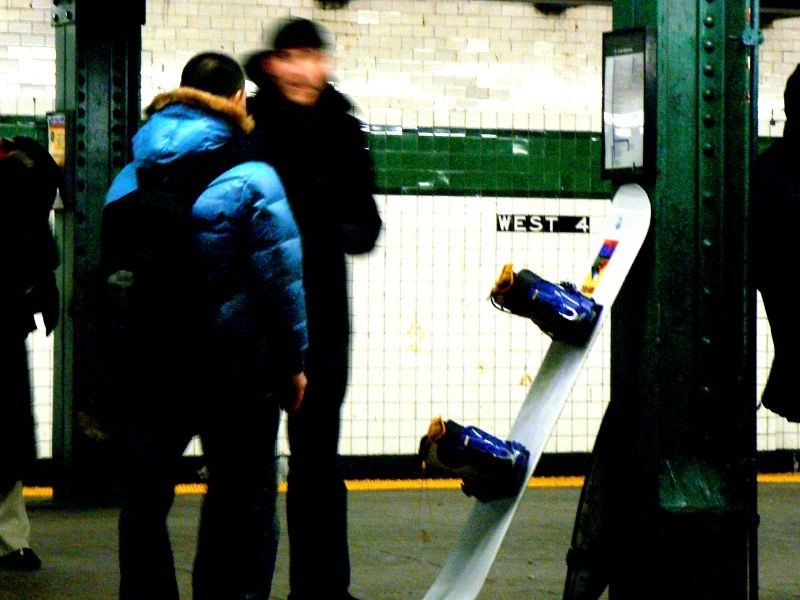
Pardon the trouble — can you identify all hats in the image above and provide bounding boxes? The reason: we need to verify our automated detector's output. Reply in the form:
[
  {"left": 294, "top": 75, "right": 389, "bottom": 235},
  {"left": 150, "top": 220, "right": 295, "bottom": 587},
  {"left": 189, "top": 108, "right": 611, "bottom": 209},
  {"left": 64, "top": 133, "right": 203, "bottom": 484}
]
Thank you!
[{"left": 273, "top": 19, "right": 324, "bottom": 49}]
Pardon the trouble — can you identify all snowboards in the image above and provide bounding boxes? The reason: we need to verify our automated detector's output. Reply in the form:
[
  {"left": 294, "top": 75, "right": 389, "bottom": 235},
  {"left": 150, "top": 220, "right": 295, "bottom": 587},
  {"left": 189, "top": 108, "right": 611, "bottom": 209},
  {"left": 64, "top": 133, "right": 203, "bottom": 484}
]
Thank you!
[{"left": 420, "top": 183, "right": 652, "bottom": 600}]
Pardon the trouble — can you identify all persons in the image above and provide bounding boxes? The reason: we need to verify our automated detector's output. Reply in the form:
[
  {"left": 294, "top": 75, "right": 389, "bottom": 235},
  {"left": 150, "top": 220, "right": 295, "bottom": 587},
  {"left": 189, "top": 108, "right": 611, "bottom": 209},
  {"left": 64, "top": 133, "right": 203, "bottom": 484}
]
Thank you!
[
  {"left": 754, "top": 59, "right": 800, "bottom": 424},
  {"left": 186, "top": 14, "right": 384, "bottom": 600},
  {"left": 0, "top": 130, "right": 64, "bottom": 567},
  {"left": 98, "top": 51, "right": 309, "bottom": 600}
]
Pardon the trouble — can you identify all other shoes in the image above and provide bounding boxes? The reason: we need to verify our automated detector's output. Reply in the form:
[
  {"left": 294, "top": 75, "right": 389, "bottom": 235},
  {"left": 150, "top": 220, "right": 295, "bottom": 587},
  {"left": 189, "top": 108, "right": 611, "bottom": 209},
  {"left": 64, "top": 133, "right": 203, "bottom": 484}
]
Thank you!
[{"left": 0, "top": 548, "right": 43, "bottom": 572}]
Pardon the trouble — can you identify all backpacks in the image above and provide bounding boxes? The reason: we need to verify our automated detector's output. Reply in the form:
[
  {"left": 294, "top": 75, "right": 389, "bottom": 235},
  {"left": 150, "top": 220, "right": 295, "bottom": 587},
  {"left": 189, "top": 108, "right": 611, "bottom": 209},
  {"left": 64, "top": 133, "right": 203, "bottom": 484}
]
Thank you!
[{"left": 94, "top": 147, "right": 262, "bottom": 431}]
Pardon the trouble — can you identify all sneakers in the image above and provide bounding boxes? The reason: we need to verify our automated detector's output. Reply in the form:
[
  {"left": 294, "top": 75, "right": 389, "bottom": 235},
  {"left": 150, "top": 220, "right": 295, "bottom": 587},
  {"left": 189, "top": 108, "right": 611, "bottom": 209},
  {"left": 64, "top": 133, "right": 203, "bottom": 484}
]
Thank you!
[
  {"left": 487, "top": 263, "right": 601, "bottom": 348},
  {"left": 417, "top": 416, "right": 529, "bottom": 504}
]
